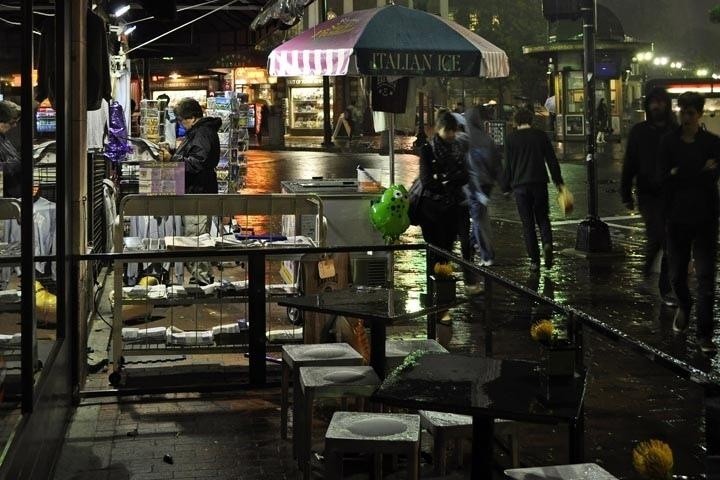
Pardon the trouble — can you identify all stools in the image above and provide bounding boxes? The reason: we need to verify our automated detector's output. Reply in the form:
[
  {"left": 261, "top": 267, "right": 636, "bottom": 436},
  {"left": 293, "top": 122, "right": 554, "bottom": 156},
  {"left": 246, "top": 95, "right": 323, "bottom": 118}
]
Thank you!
[
  {"left": 282, "top": 343, "right": 364, "bottom": 459},
  {"left": 297, "top": 366, "right": 383, "bottom": 480},
  {"left": 419, "top": 409, "right": 518, "bottom": 480},
  {"left": 504, "top": 463, "right": 619, "bottom": 480},
  {"left": 325, "top": 411, "right": 420, "bottom": 480},
  {"left": 385, "top": 339, "right": 450, "bottom": 380}
]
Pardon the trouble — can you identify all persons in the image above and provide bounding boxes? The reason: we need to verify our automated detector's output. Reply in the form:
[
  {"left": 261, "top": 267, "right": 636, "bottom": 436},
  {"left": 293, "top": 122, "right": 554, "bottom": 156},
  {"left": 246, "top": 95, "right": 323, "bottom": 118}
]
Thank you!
[
  {"left": 595, "top": 96, "right": 607, "bottom": 145},
  {"left": 158, "top": 98, "right": 222, "bottom": 194},
  {"left": 655, "top": 93, "right": 720, "bottom": 353},
  {"left": 620, "top": 89, "right": 679, "bottom": 308},
  {"left": 414, "top": 105, "right": 564, "bottom": 324}
]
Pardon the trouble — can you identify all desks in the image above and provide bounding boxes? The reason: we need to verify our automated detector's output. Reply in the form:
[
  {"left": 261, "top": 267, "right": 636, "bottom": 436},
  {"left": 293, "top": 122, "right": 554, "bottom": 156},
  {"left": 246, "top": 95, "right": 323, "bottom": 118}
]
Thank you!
[
  {"left": 278, "top": 286, "right": 466, "bottom": 376},
  {"left": 376, "top": 352, "right": 594, "bottom": 480}
]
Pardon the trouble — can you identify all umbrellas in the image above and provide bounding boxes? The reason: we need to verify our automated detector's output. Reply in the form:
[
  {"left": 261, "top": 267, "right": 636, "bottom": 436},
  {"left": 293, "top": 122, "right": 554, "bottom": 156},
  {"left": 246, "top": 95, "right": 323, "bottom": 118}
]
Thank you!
[
  {"left": 545, "top": 95, "right": 556, "bottom": 114},
  {"left": 267, "top": 4, "right": 510, "bottom": 282}
]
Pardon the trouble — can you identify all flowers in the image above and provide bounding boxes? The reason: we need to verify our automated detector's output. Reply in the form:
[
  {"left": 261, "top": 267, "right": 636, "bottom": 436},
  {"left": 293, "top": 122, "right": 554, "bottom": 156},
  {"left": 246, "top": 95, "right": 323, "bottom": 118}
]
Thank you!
[
  {"left": 632, "top": 439, "right": 673, "bottom": 480},
  {"left": 530, "top": 320, "right": 554, "bottom": 351},
  {"left": 434, "top": 261, "right": 453, "bottom": 278}
]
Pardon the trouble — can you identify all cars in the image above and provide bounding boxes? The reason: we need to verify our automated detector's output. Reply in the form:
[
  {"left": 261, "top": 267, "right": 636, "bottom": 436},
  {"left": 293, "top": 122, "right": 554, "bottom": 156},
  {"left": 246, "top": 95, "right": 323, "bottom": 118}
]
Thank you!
[{"left": 461, "top": 104, "right": 542, "bottom": 130}]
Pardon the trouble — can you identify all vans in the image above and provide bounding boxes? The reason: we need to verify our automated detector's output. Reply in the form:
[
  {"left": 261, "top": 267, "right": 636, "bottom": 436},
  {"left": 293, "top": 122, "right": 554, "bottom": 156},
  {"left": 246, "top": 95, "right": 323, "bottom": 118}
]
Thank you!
[{"left": 416, "top": 104, "right": 451, "bottom": 126}]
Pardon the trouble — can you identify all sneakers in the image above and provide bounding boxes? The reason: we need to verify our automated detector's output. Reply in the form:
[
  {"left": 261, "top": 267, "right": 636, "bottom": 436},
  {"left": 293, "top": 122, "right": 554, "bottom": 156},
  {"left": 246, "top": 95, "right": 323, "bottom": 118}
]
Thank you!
[
  {"left": 662, "top": 293, "right": 678, "bottom": 306},
  {"left": 531, "top": 261, "right": 540, "bottom": 270},
  {"left": 699, "top": 339, "right": 716, "bottom": 353},
  {"left": 478, "top": 258, "right": 494, "bottom": 267},
  {"left": 544, "top": 243, "right": 553, "bottom": 262},
  {"left": 673, "top": 308, "right": 690, "bottom": 331}
]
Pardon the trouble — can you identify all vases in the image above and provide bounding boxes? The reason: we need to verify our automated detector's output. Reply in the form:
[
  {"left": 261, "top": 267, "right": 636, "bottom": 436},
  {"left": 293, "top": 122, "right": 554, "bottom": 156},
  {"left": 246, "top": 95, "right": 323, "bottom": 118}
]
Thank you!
[{"left": 430, "top": 275, "right": 456, "bottom": 302}]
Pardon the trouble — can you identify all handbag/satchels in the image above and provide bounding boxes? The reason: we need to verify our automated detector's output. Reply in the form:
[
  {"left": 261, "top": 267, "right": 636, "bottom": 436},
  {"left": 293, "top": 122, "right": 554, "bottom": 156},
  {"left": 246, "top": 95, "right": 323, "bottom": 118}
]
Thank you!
[{"left": 406, "top": 177, "right": 431, "bottom": 225}]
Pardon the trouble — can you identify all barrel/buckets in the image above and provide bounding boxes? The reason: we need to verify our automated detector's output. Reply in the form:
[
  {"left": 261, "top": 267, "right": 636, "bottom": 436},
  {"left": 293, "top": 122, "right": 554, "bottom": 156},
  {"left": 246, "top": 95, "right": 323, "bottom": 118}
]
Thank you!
[{"left": 357, "top": 167, "right": 382, "bottom": 191}]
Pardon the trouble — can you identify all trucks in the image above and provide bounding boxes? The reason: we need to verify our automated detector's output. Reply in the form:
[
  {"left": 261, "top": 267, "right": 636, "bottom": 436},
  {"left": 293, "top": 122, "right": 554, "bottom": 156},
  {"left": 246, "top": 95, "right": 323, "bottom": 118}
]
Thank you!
[
  {"left": 34, "top": 106, "right": 57, "bottom": 136},
  {"left": 179, "top": 89, "right": 256, "bottom": 136}
]
